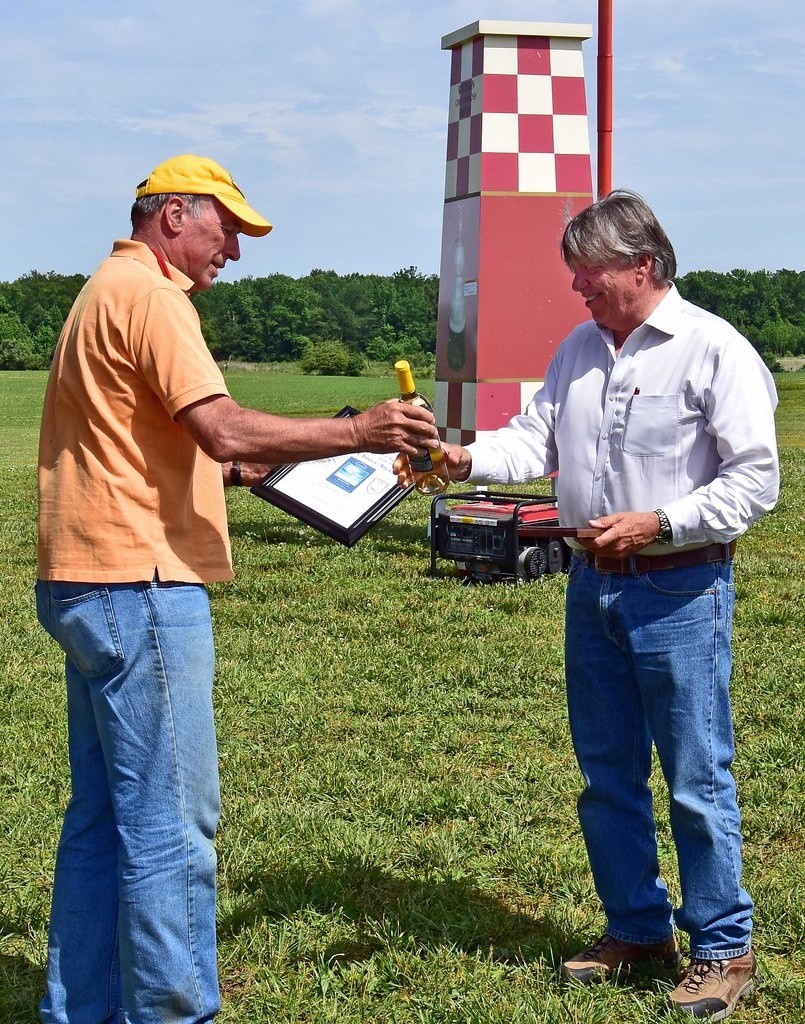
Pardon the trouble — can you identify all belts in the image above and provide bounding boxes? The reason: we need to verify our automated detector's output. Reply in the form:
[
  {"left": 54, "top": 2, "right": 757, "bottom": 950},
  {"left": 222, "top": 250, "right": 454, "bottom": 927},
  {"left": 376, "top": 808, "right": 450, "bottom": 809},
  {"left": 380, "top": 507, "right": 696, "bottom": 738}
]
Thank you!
[{"left": 572, "top": 538, "right": 737, "bottom": 575}]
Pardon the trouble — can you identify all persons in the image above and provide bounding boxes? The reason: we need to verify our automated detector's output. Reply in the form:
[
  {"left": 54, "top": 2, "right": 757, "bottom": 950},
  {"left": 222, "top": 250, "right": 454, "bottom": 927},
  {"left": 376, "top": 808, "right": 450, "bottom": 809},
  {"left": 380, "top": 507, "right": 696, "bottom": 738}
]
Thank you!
[
  {"left": 34, "top": 154, "right": 438, "bottom": 1023},
  {"left": 394, "top": 191, "right": 780, "bottom": 1024}
]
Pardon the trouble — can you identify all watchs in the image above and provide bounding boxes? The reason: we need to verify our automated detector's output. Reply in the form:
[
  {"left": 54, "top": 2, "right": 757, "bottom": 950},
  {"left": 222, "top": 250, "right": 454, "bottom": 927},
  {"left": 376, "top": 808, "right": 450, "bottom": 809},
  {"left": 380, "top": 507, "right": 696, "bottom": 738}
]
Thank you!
[{"left": 654, "top": 509, "right": 673, "bottom": 545}]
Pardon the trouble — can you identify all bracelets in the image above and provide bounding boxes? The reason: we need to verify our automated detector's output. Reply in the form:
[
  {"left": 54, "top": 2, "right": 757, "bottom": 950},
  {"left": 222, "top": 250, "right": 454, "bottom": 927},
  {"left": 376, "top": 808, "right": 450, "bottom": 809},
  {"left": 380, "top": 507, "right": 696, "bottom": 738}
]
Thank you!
[{"left": 231, "top": 460, "right": 243, "bottom": 487}]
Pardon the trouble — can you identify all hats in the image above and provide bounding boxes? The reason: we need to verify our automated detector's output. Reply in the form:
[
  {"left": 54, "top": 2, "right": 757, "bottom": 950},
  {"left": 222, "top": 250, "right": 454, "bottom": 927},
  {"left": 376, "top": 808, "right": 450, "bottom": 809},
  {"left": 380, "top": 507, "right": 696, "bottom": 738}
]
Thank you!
[{"left": 135, "top": 154, "right": 273, "bottom": 237}]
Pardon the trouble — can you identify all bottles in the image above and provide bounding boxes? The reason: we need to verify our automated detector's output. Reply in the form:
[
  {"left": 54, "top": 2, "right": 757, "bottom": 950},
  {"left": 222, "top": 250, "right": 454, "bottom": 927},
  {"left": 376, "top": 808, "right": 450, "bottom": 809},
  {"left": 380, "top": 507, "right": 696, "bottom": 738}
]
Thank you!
[{"left": 395, "top": 360, "right": 450, "bottom": 496}]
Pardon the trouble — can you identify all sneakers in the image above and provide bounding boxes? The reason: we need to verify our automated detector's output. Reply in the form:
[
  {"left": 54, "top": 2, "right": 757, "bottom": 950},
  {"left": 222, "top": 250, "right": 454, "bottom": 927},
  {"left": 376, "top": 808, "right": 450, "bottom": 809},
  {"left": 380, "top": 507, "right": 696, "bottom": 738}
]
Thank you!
[
  {"left": 563, "top": 934, "right": 680, "bottom": 986},
  {"left": 668, "top": 949, "right": 757, "bottom": 1023}
]
